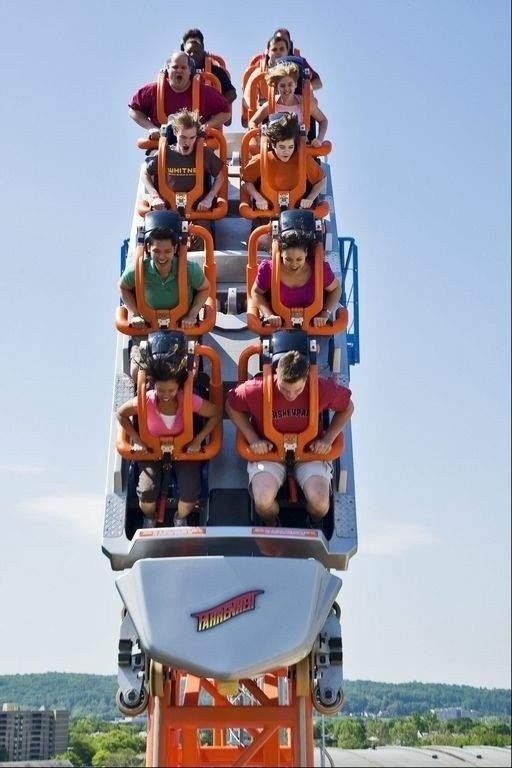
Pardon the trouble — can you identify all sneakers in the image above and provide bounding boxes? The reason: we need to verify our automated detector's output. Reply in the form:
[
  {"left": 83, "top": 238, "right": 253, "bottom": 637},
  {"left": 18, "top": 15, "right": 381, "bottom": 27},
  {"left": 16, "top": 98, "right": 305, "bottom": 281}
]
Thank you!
[
  {"left": 172, "top": 511, "right": 187, "bottom": 527},
  {"left": 142, "top": 513, "right": 156, "bottom": 528}
]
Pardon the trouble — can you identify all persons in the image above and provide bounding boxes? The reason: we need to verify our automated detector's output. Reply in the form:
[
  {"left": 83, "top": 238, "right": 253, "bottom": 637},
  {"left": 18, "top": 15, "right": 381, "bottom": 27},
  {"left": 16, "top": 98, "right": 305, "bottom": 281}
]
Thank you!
[
  {"left": 251, "top": 229, "right": 344, "bottom": 381},
  {"left": 242, "top": 112, "right": 329, "bottom": 251},
  {"left": 274, "top": 28, "right": 294, "bottom": 55},
  {"left": 248, "top": 64, "right": 330, "bottom": 149},
  {"left": 116, "top": 225, "right": 212, "bottom": 397},
  {"left": 226, "top": 349, "right": 355, "bottom": 539},
  {"left": 116, "top": 358, "right": 223, "bottom": 530},
  {"left": 184, "top": 39, "right": 239, "bottom": 103},
  {"left": 266, "top": 34, "right": 323, "bottom": 95},
  {"left": 183, "top": 28, "right": 205, "bottom": 51},
  {"left": 129, "top": 50, "right": 233, "bottom": 139},
  {"left": 141, "top": 104, "right": 229, "bottom": 251}
]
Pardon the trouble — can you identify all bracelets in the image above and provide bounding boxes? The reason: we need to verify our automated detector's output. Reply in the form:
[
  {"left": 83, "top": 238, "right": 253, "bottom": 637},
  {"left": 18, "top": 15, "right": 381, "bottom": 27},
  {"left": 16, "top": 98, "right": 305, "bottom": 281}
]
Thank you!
[{"left": 322, "top": 308, "right": 332, "bottom": 316}]
